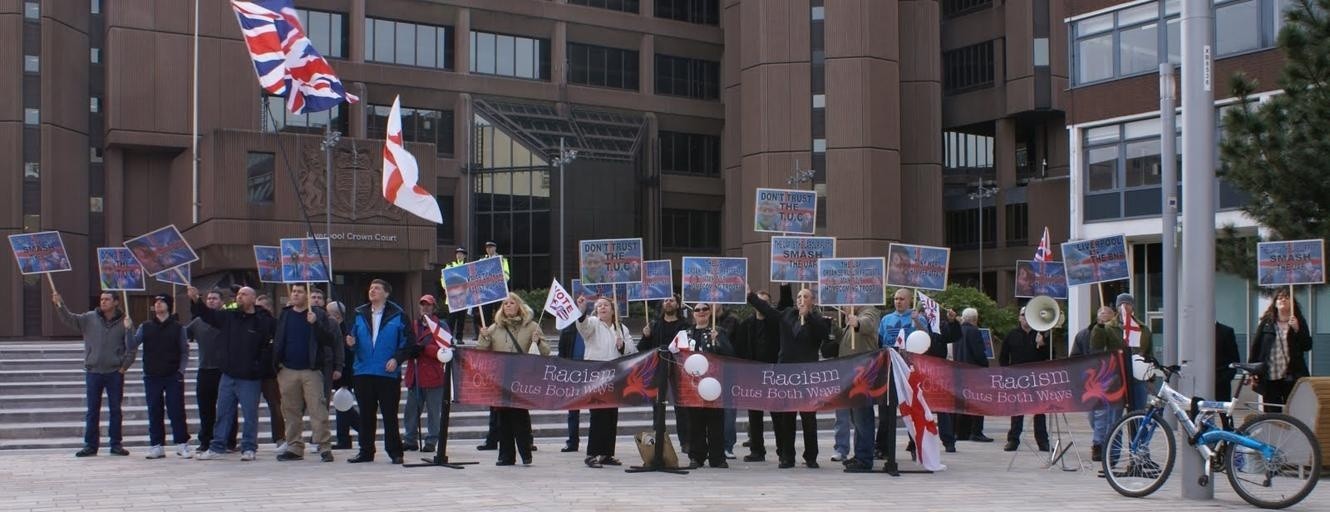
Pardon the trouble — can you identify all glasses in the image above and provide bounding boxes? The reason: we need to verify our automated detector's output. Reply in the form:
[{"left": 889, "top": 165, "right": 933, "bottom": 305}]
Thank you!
[{"left": 693, "top": 304, "right": 711, "bottom": 316}]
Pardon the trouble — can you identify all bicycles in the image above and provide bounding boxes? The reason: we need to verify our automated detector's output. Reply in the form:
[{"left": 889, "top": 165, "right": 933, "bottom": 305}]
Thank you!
[{"left": 1101, "top": 353, "right": 1320, "bottom": 508}]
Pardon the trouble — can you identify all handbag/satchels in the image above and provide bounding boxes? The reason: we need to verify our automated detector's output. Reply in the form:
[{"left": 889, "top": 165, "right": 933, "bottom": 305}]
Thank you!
[
  {"left": 634, "top": 430, "right": 679, "bottom": 468},
  {"left": 1282, "top": 365, "right": 1311, "bottom": 392}
]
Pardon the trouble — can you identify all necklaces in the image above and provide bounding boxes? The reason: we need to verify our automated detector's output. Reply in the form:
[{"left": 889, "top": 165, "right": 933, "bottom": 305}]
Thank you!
[{"left": 1279, "top": 319, "right": 1291, "bottom": 323}]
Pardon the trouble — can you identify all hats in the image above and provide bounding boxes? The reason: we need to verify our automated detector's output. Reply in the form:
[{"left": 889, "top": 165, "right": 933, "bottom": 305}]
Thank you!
[
  {"left": 484, "top": 239, "right": 498, "bottom": 247},
  {"left": 454, "top": 245, "right": 468, "bottom": 254},
  {"left": 418, "top": 291, "right": 438, "bottom": 309},
  {"left": 1114, "top": 291, "right": 1137, "bottom": 310},
  {"left": 151, "top": 291, "right": 177, "bottom": 308}
]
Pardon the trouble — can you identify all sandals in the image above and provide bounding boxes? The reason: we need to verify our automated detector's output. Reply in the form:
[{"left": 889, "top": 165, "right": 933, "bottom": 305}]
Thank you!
[
  {"left": 598, "top": 454, "right": 623, "bottom": 467},
  {"left": 584, "top": 455, "right": 605, "bottom": 470}
]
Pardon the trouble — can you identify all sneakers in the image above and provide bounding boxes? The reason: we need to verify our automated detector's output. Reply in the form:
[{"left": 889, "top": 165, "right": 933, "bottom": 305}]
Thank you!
[
  {"left": 678, "top": 436, "right": 894, "bottom": 473},
  {"left": 521, "top": 453, "right": 534, "bottom": 467},
  {"left": 942, "top": 430, "right": 996, "bottom": 453},
  {"left": 473, "top": 436, "right": 498, "bottom": 451},
  {"left": 1003, "top": 437, "right": 1019, "bottom": 451},
  {"left": 450, "top": 335, "right": 465, "bottom": 345},
  {"left": 73, "top": 437, "right": 443, "bottom": 465},
  {"left": 1037, "top": 442, "right": 1055, "bottom": 453},
  {"left": 559, "top": 442, "right": 580, "bottom": 453},
  {"left": 1143, "top": 458, "right": 1161, "bottom": 470},
  {"left": 909, "top": 448, "right": 918, "bottom": 463},
  {"left": 1095, "top": 460, "right": 1117, "bottom": 480},
  {"left": 471, "top": 335, "right": 477, "bottom": 341},
  {"left": 494, "top": 457, "right": 515, "bottom": 468},
  {"left": 528, "top": 444, "right": 538, "bottom": 452},
  {"left": 1090, "top": 444, "right": 1103, "bottom": 461}
]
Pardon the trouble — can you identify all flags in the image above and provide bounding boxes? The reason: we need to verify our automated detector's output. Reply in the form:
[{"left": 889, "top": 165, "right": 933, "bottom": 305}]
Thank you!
[
  {"left": 543, "top": 277, "right": 583, "bottom": 331},
  {"left": 382, "top": 92, "right": 444, "bottom": 230},
  {"left": 1034, "top": 227, "right": 1055, "bottom": 263},
  {"left": 231, "top": 0, "right": 360, "bottom": 117}
]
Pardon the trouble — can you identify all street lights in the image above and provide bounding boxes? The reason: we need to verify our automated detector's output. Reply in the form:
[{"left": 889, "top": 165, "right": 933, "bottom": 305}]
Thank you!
[
  {"left": 968, "top": 177, "right": 1001, "bottom": 293},
  {"left": 321, "top": 108, "right": 341, "bottom": 300},
  {"left": 552, "top": 136, "right": 578, "bottom": 291},
  {"left": 783, "top": 159, "right": 817, "bottom": 192},
  {"left": 1158, "top": 60, "right": 1182, "bottom": 431}
]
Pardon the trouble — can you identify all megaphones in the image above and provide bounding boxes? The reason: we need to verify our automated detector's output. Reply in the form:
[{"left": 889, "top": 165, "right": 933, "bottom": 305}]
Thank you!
[{"left": 1024, "top": 296, "right": 1066, "bottom": 336}]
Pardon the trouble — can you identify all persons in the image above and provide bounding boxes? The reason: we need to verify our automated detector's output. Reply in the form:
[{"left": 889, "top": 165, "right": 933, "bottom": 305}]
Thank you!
[
  {"left": 479, "top": 240, "right": 511, "bottom": 328},
  {"left": 441, "top": 246, "right": 467, "bottom": 345},
  {"left": 1215, "top": 322, "right": 1240, "bottom": 448},
  {"left": 820, "top": 286, "right": 1153, "bottom": 472},
  {"left": 51, "top": 287, "right": 455, "bottom": 464},
  {"left": 476, "top": 287, "right": 832, "bottom": 469},
  {"left": 1248, "top": 287, "right": 1312, "bottom": 414}
]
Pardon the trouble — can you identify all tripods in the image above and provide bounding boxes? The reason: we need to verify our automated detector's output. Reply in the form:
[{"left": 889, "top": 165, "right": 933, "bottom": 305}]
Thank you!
[{"left": 1005, "top": 333, "right": 1098, "bottom": 479}]
